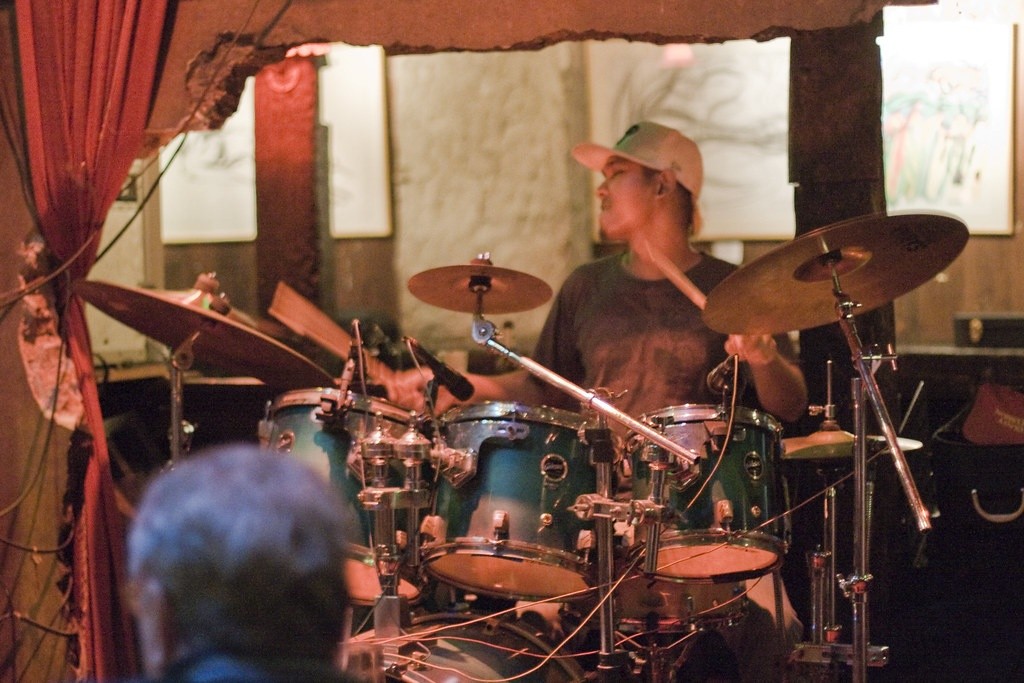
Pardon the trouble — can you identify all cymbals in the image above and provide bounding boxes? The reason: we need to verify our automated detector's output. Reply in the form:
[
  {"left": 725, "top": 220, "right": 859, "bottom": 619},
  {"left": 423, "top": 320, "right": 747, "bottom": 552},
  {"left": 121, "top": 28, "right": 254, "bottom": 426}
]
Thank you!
[
  {"left": 406, "top": 263, "right": 553, "bottom": 315},
  {"left": 139, "top": 272, "right": 257, "bottom": 327},
  {"left": 77, "top": 271, "right": 333, "bottom": 387},
  {"left": 700, "top": 214, "right": 970, "bottom": 336},
  {"left": 782, "top": 420, "right": 923, "bottom": 459}
]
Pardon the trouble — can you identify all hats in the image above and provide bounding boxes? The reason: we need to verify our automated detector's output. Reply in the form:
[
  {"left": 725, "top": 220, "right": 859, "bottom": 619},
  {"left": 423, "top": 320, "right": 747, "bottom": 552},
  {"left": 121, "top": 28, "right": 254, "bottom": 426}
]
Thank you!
[{"left": 570, "top": 121, "right": 703, "bottom": 198}]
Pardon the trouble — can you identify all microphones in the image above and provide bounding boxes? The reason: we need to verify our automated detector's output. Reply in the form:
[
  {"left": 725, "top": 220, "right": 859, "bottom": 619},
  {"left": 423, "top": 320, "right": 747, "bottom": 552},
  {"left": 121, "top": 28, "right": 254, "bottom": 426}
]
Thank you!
[
  {"left": 402, "top": 336, "right": 474, "bottom": 403},
  {"left": 348, "top": 320, "right": 366, "bottom": 388},
  {"left": 707, "top": 353, "right": 739, "bottom": 394}
]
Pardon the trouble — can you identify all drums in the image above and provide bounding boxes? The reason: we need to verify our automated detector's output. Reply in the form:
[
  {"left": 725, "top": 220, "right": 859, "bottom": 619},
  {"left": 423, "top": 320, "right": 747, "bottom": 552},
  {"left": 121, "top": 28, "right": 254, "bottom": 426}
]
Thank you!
[
  {"left": 258, "top": 386, "right": 428, "bottom": 604},
  {"left": 613, "top": 546, "right": 749, "bottom": 633},
  {"left": 423, "top": 401, "right": 596, "bottom": 602},
  {"left": 624, "top": 404, "right": 789, "bottom": 584},
  {"left": 343, "top": 612, "right": 735, "bottom": 682}
]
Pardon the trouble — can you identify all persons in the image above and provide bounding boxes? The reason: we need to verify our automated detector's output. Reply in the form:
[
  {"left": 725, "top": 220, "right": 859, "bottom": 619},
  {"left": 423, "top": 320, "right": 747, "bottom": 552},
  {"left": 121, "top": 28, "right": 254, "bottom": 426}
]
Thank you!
[
  {"left": 387, "top": 120, "right": 807, "bottom": 504},
  {"left": 129, "top": 447, "right": 377, "bottom": 683}
]
